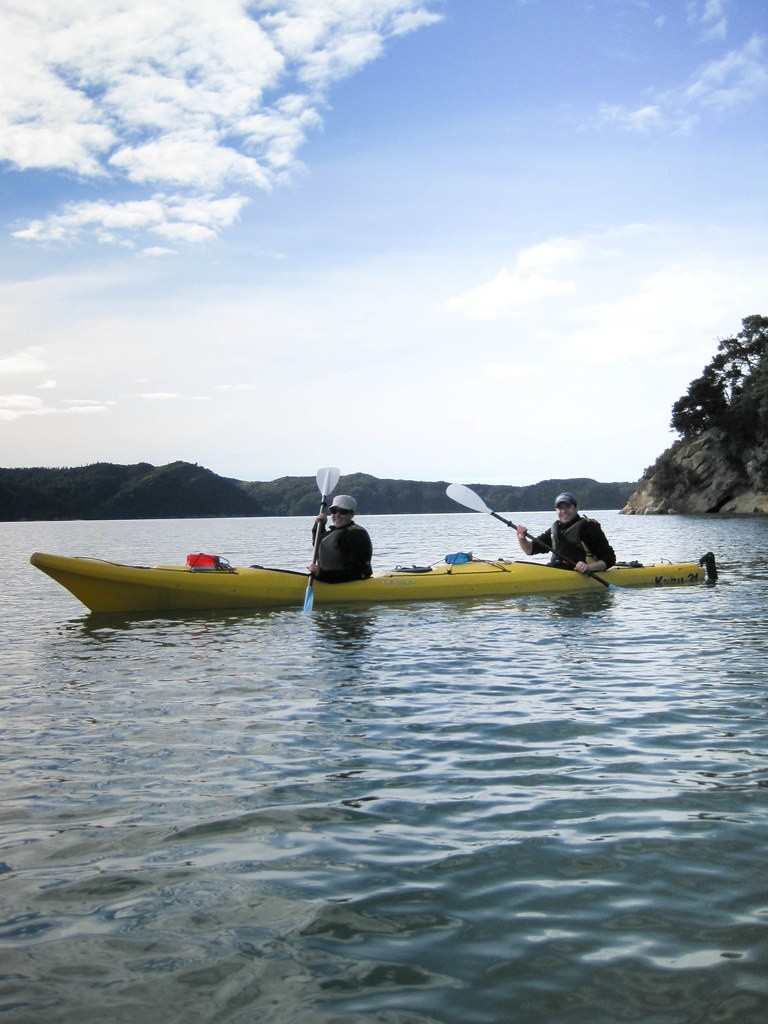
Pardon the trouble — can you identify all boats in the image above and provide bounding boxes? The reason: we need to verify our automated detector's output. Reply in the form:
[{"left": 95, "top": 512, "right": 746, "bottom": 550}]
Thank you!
[{"left": 29, "top": 551, "right": 718, "bottom": 611}]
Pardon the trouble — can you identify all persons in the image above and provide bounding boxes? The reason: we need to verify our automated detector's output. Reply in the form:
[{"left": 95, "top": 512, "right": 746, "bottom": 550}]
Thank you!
[
  {"left": 516, "top": 492, "right": 616, "bottom": 574},
  {"left": 306, "top": 495, "right": 373, "bottom": 584}
]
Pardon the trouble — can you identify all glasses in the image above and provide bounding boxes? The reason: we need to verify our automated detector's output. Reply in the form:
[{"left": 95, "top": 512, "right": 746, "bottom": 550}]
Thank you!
[{"left": 330, "top": 509, "right": 351, "bottom": 514}]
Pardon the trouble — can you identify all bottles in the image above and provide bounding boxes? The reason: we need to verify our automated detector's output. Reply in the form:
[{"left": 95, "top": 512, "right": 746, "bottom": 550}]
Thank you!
[{"left": 445, "top": 553, "right": 472, "bottom": 565}]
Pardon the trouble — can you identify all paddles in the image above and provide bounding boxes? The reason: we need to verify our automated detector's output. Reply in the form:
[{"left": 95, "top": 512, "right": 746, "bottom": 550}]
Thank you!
[
  {"left": 302, "top": 466, "right": 341, "bottom": 614},
  {"left": 443, "top": 482, "right": 627, "bottom": 591}
]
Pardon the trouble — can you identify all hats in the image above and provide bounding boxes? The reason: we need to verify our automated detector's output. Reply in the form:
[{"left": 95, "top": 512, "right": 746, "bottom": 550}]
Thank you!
[
  {"left": 555, "top": 492, "right": 577, "bottom": 508},
  {"left": 328, "top": 495, "right": 357, "bottom": 512}
]
